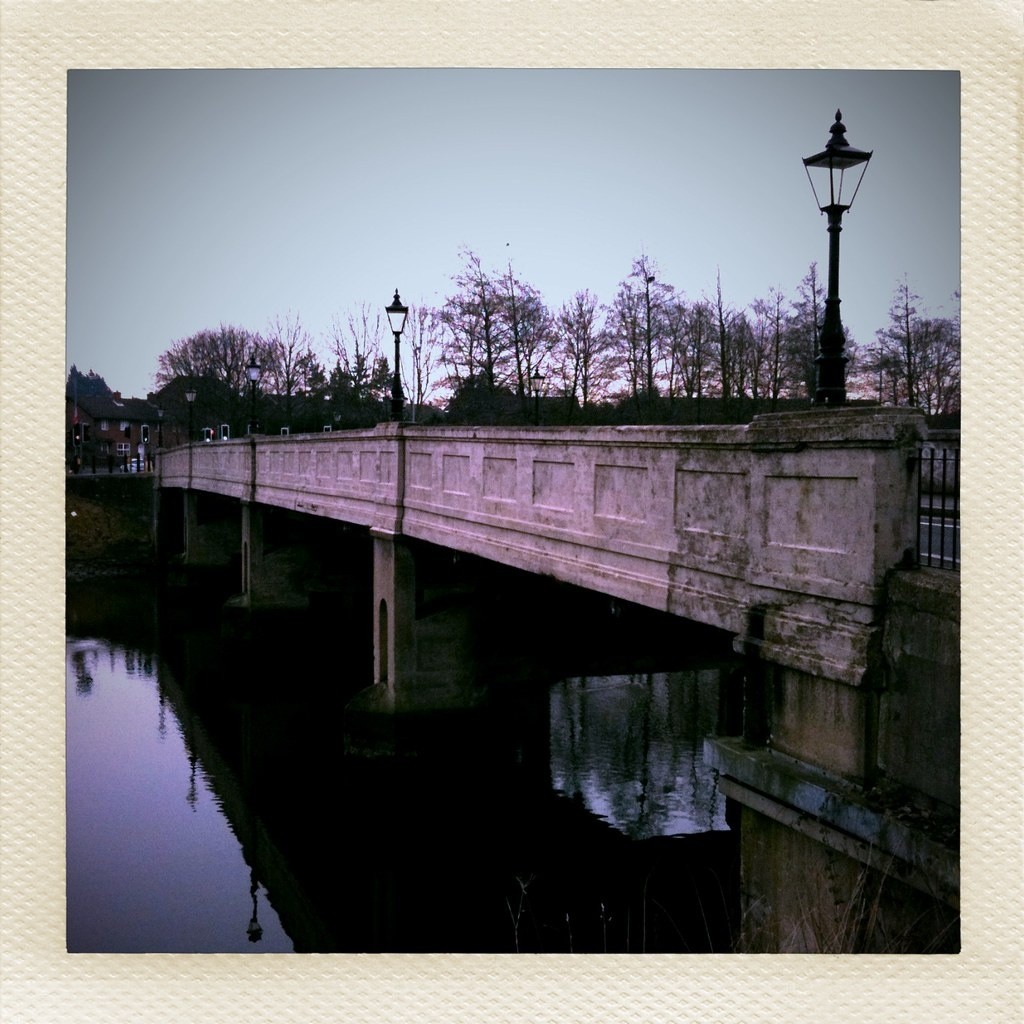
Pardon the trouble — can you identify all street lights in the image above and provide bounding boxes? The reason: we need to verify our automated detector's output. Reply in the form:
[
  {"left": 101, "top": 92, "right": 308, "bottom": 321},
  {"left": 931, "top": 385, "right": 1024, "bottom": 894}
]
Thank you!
[
  {"left": 384, "top": 287, "right": 411, "bottom": 423},
  {"left": 157, "top": 403, "right": 166, "bottom": 447},
  {"left": 185, "top": 384, "right": 197, "bottom": 442},
  {"left": 245, "top": 355, "right": 262, "bottom": 433},
  {"left": 803, "top": 109, "right": 875, "bottom": 407}
]
